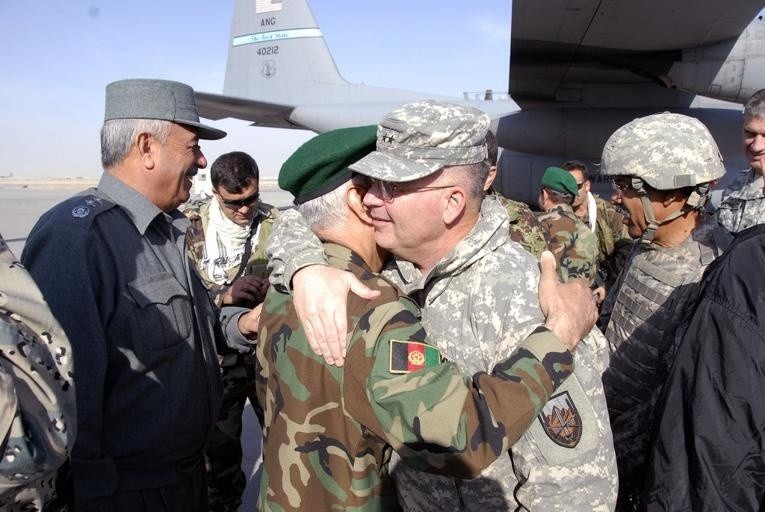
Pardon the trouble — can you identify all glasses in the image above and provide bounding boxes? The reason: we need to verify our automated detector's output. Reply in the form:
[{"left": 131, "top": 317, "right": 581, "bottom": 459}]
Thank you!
[
  {"left": 577, "top": 182, "right": 584, "bottom": 189},
  {"left": 348, "top": 100, "right": 491, "bottom": 182},
  {"left": 217, "top": 177, "right": 259, "bottom": 205},
  {"left": 611, "top": 180, "right": 649, "bottom": 197},
  {"left": 374, "top": 178, "right": 455, "bottom": 203}
]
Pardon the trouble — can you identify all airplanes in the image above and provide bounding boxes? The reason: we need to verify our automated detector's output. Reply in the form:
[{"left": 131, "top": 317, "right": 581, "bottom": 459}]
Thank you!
[{"left": 191, "top": 1, "right": 765, "bottom": 219}]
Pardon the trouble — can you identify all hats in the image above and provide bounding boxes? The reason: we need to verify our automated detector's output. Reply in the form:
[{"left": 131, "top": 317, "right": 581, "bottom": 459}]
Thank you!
[
  {"left": 104, "top": 77, "right": 228, "bottom": 140},
  {"left": 541, "top": 166, "right": 579, "bottom": 197},
  {"left": 278, "top": 125, "right": 377, "bottom": 205}
]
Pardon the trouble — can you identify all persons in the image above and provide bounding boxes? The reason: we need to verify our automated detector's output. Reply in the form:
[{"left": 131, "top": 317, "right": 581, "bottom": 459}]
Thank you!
[
  {"left": 562, "top": 160, "right": 633, "bottom": 303},
  {"left": 646, "top": 224, "right": 764, "bottom": 512},
  {"left": 537, "top": 168, "right": 600, "bottom": 289},
  {"left": 483, "top": 129, "right": 563, "bottom": 280},
  {"left": 255, "top": 126, "right": 598, "bottom": 511},
  {"left": 266, "top": 99, "right": 619, "bottom": 511},
  {"left": 20, "top": 79, "right": 264, "bottom": 511},
  {"left": 598, "top": 113, "right": 727, "bottom": 512},
  {"left": 1, "top": 237, "right": 76, "bottom": 512},
  {"left": 182, "top": 151, "right": 279, "bottom": 512}
]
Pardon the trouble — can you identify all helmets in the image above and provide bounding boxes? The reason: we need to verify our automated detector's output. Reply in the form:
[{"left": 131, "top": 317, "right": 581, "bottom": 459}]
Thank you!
[{"left": 600, "top": 111, "right": 726, "bottom": 190}]
